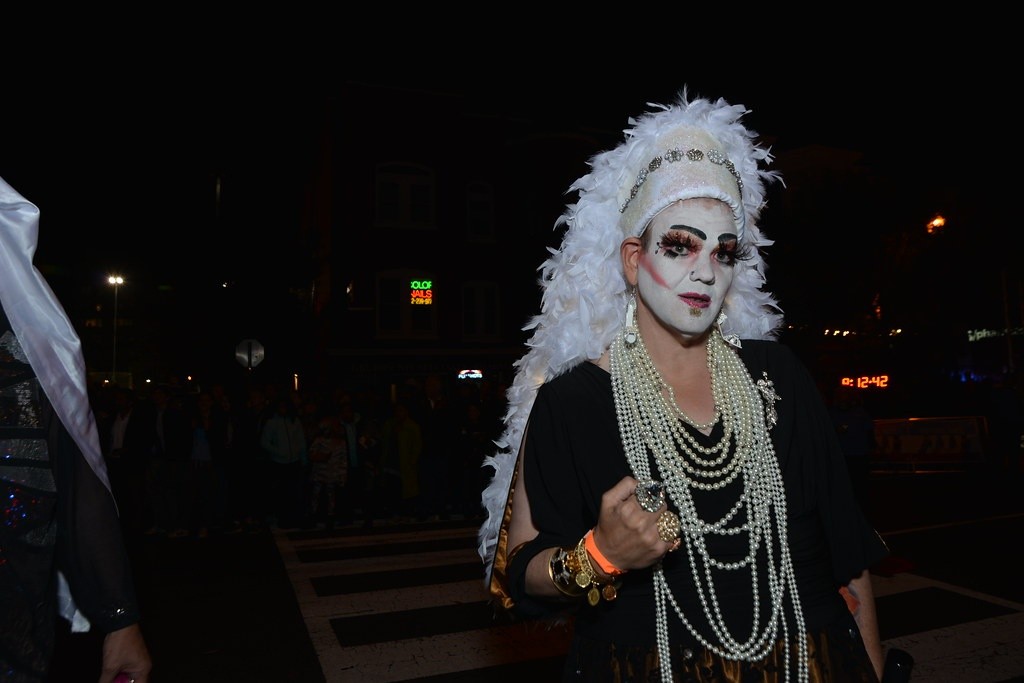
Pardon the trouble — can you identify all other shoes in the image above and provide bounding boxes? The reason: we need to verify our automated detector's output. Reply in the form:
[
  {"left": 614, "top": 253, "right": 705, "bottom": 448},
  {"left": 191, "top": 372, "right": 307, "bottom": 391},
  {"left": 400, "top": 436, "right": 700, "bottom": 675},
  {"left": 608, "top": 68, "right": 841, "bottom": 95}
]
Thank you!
[
  {"left": 387, "top": 514, "right": 416, "bottom": 527},
  {"left": 223, "top": 525, "right": 243, "bottom": 534},
  {"left": 167, "top": 528, "right": 190, "bottom": 538},
  {"left": 144, "top": 527, "right": 165, "bottom": 534},
  {"left": 197, "top": 527, "right": 208, "bottom": 538}
]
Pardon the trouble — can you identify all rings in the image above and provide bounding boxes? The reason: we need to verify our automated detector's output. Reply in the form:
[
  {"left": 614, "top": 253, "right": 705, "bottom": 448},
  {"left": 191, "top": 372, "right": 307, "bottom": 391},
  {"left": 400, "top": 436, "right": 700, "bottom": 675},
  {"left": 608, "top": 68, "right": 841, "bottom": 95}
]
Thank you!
[
  {"left": 658, "top": 511, "right": 680, "bottom": 540},
  {"left": 670, "top": 538, "right": 681, "bottom": 551},
  {"left": 636, "top": 479, "right": 666, "bottom": 512}
]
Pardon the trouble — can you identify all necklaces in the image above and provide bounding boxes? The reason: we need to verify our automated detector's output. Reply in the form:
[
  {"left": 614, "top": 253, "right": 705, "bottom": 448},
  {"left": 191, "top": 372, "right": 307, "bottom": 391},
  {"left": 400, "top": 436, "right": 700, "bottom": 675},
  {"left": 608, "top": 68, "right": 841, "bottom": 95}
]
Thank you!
[{"left": 611, "top": 321, "right": 809, "bottom": 683}]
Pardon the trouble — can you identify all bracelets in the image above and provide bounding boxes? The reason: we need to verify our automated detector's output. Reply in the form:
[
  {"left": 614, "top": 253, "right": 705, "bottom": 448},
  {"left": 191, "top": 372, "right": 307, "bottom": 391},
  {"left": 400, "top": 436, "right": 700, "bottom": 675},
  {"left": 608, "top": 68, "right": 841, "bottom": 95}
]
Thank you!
[{"left": 547, "top": 529, "right": 626, "bottom": 605}]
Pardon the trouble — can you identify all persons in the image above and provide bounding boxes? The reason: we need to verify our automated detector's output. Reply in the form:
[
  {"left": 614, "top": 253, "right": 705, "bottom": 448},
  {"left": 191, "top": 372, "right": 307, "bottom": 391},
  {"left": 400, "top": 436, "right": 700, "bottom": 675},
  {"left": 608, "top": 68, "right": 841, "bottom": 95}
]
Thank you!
[
  {"left": 109, "top": 371, "right": 422, "bottom": 534},
  {"left": 0, "top": 172, "right": 153, "bottom": 683},
  {"left": 488, "top": 99, "right": 883, "bottom": 683}
]
